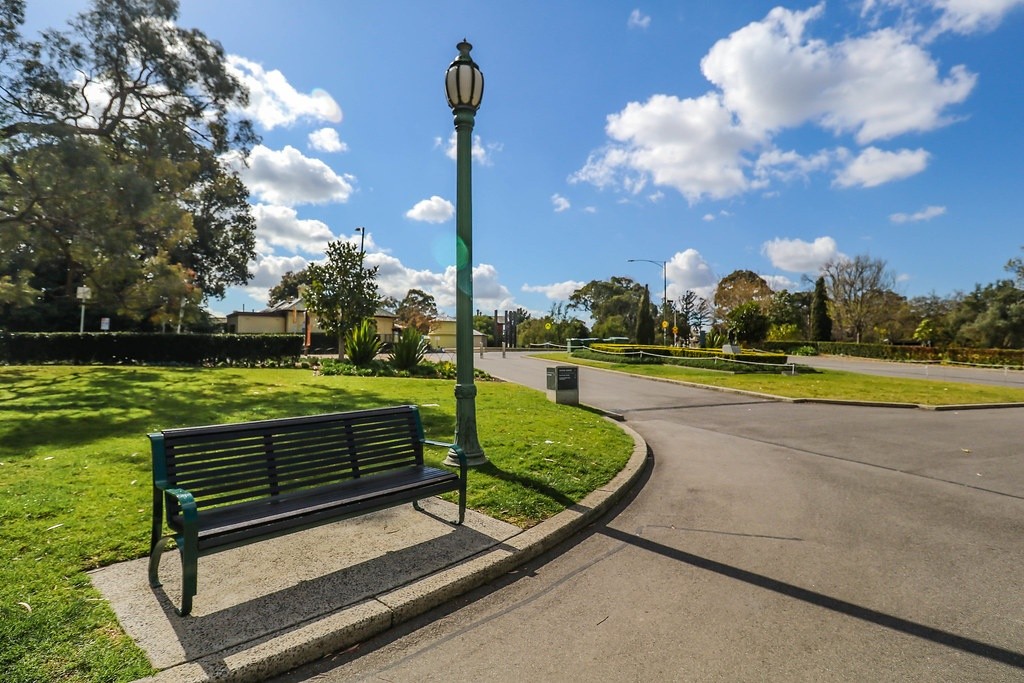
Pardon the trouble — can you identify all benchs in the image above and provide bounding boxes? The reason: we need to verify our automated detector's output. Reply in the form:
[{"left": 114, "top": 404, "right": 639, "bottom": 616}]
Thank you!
[{"left": 146, "top": 405, "right": 467, "bottom": 617}]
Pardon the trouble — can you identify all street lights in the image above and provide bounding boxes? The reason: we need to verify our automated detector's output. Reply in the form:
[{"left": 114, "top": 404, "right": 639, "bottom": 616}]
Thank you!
[
  {"left": 627, "top": 259, "right": 668, "bottom": 346},
  {"left": 441, "top": 37, "right": 494, "bottom": 471},
  {"left": 667, "top": 299, "right": 677, "bottom": 347},
  {"left": 354, "top": 226, "right": 366, "bottom": 330}
]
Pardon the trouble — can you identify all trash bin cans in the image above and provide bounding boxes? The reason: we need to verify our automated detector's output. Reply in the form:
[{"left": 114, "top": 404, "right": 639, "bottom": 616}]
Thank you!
[{"left": 546, "top": 365, "right": 580, "bottom": 405}]
